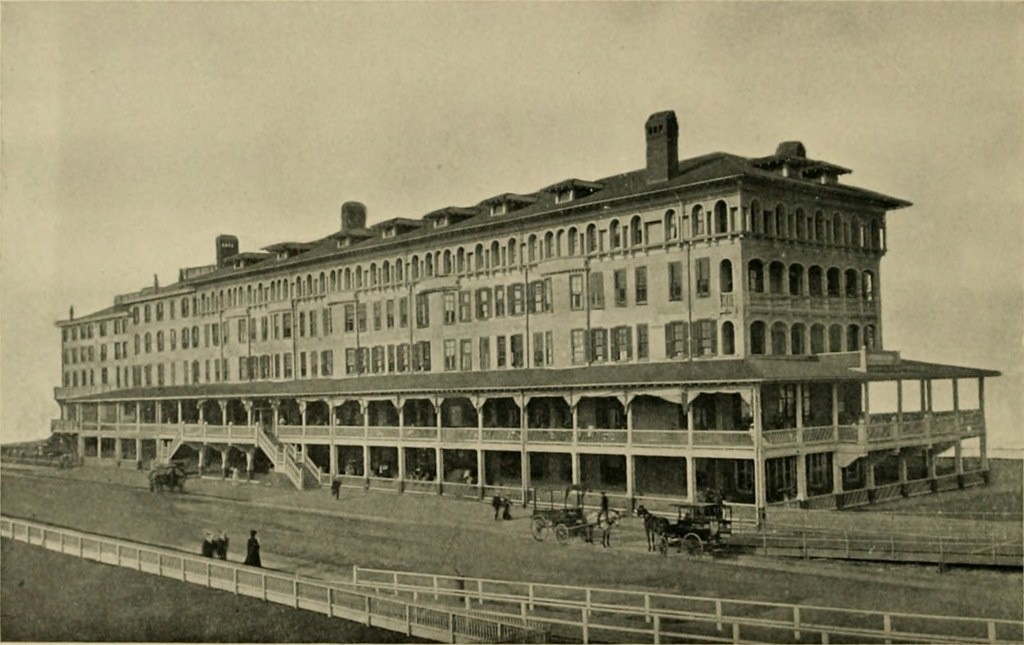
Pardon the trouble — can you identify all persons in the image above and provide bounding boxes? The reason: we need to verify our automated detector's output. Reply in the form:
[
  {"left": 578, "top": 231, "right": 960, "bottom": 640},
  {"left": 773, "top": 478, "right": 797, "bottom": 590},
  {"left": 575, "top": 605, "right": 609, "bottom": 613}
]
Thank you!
[
  {"left": 202, "top": 529, "right": 229, "bottom": 560},
  {"left": 705, "top": 485, "right": 727, "bottom": 503},
  {"left": 376, "top": 465, "right": 384, "bottom": 475},
  {"left": 331, "top": 475, "right": 341, "bottom": 499},
  {"left": 493, "top": 491, "right": 513, "bottom": 520},
  {"left": 413, "top": 469, "right": 433, "bottom": 481},
  {"left": 232, "top": 466, "right": 240, "bottom": 483},
  {"left": 137, "top": 460, "right": 142, "bottom": 473},
  {"left": 597, "top": 491, "right": 608, "bottom": 526},
  {"left": 631, "top": 495, "right": 636, "bottom": 512},
  {"left": 278, "top": 415, "right": 286, "bottom": 425},
  {"left": 741, "top": 413, "right": 753, "bottom": 430},
  {"left": 244, "top": 530, "right": 260, "bottom": 567}
]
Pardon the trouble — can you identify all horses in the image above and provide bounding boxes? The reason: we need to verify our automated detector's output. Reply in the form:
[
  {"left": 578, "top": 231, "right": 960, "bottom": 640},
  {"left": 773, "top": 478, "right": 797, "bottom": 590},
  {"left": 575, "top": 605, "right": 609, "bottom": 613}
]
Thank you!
[
  {"left": 581, "top": 508, "right": 620, "bottom": 548},
  {"left": 636, "top": 504, "right": 671, "bottom": 552}
]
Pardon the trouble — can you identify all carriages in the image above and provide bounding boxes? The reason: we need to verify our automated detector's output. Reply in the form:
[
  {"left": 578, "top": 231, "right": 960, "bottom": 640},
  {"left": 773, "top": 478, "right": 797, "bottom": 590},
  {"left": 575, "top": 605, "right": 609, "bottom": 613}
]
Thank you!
[
  {"left": 635, "top": 500, "right": 733, "bottom": 562},
  {"left": 528, "top": 484, "right": 622, "bottom": 548},
  {"left": 148, "top": 463, "right": 187, "bottom": 494}
]
